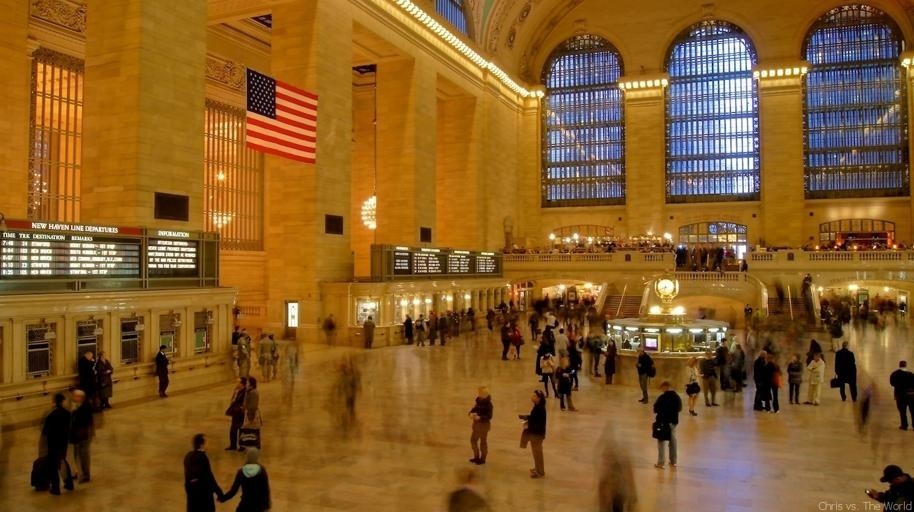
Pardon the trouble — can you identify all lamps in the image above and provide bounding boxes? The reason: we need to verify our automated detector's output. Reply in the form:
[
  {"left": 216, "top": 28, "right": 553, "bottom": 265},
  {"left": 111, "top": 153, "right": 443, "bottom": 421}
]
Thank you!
[{"left": 360, "top": 66, "right": 378, "bottom": 231}]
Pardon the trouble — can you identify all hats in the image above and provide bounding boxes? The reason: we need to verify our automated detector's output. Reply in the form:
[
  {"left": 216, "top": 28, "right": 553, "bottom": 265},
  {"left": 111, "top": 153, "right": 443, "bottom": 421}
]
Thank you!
[{"left": 879, "top": 466, "right": 901, "bottom": 483}]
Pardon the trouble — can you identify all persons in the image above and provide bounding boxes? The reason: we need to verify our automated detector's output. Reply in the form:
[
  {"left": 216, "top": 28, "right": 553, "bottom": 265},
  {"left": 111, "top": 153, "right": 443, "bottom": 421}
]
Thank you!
[{"left": 30, "top": 227, "right": 914, "bottom": 511}]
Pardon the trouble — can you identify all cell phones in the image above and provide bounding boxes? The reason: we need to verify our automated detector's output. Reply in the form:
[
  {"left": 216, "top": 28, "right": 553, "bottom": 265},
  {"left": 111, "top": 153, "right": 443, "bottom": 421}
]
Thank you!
[{"left": 864, "top": 489, "right": 873, "bottom": 498}]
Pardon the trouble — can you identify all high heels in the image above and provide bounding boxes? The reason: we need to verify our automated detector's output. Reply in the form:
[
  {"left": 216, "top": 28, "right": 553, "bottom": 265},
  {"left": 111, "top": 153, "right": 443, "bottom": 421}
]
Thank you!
[{"left": 689, "top": 409, "right": 697, "bottom": 416}]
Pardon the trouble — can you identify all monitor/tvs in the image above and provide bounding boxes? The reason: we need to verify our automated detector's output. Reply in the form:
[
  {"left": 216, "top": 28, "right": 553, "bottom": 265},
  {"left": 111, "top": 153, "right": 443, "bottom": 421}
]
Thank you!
[{"left": 644, "top": 338, "right": 658, "bottom": 348}]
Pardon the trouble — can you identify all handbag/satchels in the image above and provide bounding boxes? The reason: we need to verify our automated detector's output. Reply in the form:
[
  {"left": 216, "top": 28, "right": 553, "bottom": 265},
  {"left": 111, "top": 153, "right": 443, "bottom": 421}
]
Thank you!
[
  {"left": 830, "top": 373, "right": 839, "bottom": 387},
  {"left": 686, "top": 379, "right": 700, "bottom": 394},
  {"left": 239, "top": 426, "right": 261, "bottom": 449},
  {"left": 651, "top": 422, "right": 670, "bottom": 441}
]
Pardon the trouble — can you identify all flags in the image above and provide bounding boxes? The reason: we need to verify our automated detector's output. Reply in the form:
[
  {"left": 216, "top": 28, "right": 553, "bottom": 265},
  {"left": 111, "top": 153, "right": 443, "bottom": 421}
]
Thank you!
[{"left": 242, "top": 66, "right": 322, "bottom": 165}]
